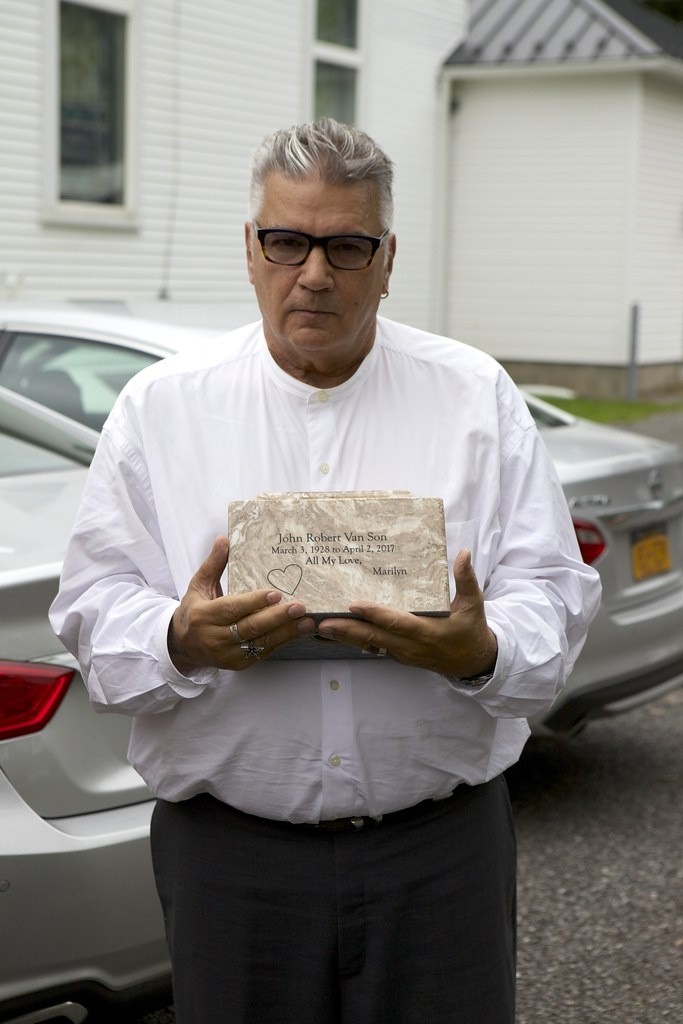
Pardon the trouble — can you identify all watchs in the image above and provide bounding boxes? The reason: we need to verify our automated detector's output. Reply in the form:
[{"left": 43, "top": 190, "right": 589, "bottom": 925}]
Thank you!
[{"left": 448, "top": 674, "right": 493, "bottom": 690}]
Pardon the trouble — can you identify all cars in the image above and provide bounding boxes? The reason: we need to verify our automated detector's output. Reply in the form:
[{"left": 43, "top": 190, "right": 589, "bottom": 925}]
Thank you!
[
  {"left": 0, "top": 305, "right": 683, "bottom": 757},
  {"left": 0, "top": 386, "right": 172, "bottom": 1024}
]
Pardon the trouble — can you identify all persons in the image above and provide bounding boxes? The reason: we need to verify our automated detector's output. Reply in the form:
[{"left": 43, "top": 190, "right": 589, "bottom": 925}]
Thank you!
[{"left": 46, "top": 115, "right": 603, "bottom": 1024}]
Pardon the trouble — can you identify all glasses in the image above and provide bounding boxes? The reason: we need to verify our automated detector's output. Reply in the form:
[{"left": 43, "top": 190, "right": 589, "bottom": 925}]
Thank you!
[{"left": 252, "top": 220, "right": 390, "bottom": 271}]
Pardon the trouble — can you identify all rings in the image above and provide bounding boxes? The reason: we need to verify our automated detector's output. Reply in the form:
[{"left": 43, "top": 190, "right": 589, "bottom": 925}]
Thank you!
[
  {"left": 229, "top": 622, "right": 245, "bottom": 644},
  {"left": 240, "top": 642, "right": 264, "bottom": 661},
  {"left": 361, "top": 633, "right": 387, "bottom": 659}
]
